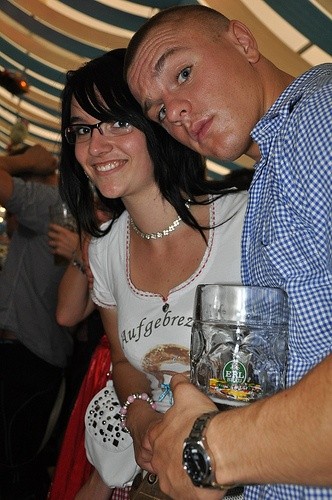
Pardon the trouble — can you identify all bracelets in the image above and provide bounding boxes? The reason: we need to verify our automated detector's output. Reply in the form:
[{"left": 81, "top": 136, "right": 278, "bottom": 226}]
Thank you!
[
  {"left": 71, "top": 258, "right": 87, "bottom": 274},
  {"left": 121, "top": 393, "right": 154, "bottom": 434}
]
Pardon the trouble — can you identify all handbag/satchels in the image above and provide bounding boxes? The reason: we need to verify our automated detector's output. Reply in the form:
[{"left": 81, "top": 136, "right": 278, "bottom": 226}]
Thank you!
[{"left": 84, "top": 380, "right": 141, "bottom": 488}]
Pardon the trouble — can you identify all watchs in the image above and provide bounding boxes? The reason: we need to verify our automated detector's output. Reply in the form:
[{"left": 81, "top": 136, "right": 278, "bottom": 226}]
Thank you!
[{"left": 181, "top": 410, "right": 240, "bottom": 491}]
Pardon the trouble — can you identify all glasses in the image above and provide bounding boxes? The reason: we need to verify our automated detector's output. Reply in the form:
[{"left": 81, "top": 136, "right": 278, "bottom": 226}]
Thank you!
[{"left": 64, "top": 118, "right": 136, "bottom": 142}]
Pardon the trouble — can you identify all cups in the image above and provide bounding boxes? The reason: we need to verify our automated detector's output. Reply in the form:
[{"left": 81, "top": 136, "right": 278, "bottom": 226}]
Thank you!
[
  {"left": 50, "top": 203, "right": 87, "bottom": 266},
  {"left": 190, "top": 284, "right": 288, "bottom": 412}
]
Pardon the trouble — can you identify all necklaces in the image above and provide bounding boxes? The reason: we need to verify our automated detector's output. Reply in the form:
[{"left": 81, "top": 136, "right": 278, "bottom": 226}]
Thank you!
[{"left": 130, "top": 199, "right": 193, "bottom": 240}]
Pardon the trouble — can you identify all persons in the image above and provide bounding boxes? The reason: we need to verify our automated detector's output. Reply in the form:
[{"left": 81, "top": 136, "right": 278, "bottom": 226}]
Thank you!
[
  {"left": 120, "top": 6, "right": 332, "bottom": 500},
  {"left": 56, "top": 48, "right": 250, "bottom": 500},
  {"left": 0, "top": 142, "right": 124, "bottom": 500}
]
